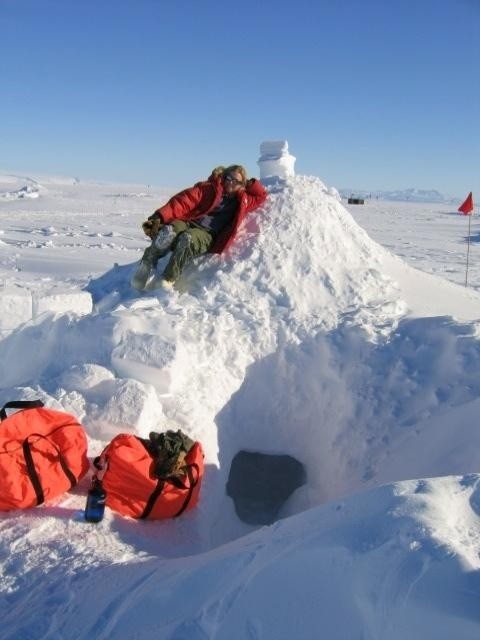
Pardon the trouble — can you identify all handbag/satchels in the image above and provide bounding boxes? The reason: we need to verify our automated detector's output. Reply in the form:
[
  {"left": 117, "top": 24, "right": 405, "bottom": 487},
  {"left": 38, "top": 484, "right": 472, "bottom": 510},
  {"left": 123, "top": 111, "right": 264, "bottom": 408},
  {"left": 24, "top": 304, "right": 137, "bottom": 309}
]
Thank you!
[
  {"left": 92, "top": 433, "right": 205, "bottom": 520},
  {"left": 0, "top": 399, "right": 91, "bottom": 512}
]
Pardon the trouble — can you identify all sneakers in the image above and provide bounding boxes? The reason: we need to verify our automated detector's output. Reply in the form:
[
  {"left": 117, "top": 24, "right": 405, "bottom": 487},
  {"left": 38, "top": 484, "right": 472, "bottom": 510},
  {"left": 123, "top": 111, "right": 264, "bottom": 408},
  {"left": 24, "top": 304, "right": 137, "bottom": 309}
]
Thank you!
[
  {"left": 133, "top": 259, "right": 155, "bottom": 289},
  {"left": 156, "top": 272, "right": 176, "bottom": 289}
]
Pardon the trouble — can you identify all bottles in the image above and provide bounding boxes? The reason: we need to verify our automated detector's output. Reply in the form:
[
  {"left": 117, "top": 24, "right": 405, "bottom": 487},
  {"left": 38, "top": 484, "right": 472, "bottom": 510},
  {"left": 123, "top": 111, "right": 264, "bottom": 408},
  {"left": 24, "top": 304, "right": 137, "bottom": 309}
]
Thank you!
[{"left": 84, "top": 474, "right": 106, "bottom": 523}]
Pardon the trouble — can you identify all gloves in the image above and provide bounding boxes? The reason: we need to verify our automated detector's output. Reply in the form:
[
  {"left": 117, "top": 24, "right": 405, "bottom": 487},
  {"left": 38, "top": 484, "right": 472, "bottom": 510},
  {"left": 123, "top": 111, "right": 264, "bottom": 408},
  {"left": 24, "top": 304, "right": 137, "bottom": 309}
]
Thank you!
[{"left": 142, "top": 212, "right": 163, "bottom": 237}]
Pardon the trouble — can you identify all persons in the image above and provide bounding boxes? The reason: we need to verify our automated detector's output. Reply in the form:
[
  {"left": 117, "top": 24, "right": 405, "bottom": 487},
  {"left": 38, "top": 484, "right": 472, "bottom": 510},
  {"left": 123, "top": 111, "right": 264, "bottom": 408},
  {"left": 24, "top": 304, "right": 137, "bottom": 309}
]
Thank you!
[{"left": 130, "top": 165, "right": 267, "bottom": 292}]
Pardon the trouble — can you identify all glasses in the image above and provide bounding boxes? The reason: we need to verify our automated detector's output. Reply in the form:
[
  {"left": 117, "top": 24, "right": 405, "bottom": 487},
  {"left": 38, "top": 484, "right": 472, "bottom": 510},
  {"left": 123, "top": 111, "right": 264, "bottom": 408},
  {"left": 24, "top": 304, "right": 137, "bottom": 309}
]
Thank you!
[{"left": 226, "top": 175, "right": 243, "bottom": 185}]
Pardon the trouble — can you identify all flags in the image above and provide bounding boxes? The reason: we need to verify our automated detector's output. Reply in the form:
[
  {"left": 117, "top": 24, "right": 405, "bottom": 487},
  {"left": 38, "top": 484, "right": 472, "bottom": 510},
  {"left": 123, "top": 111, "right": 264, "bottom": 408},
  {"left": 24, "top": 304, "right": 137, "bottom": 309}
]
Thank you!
[{"left": 457, "top": 191, "right": 473, "bottom": 216}]
